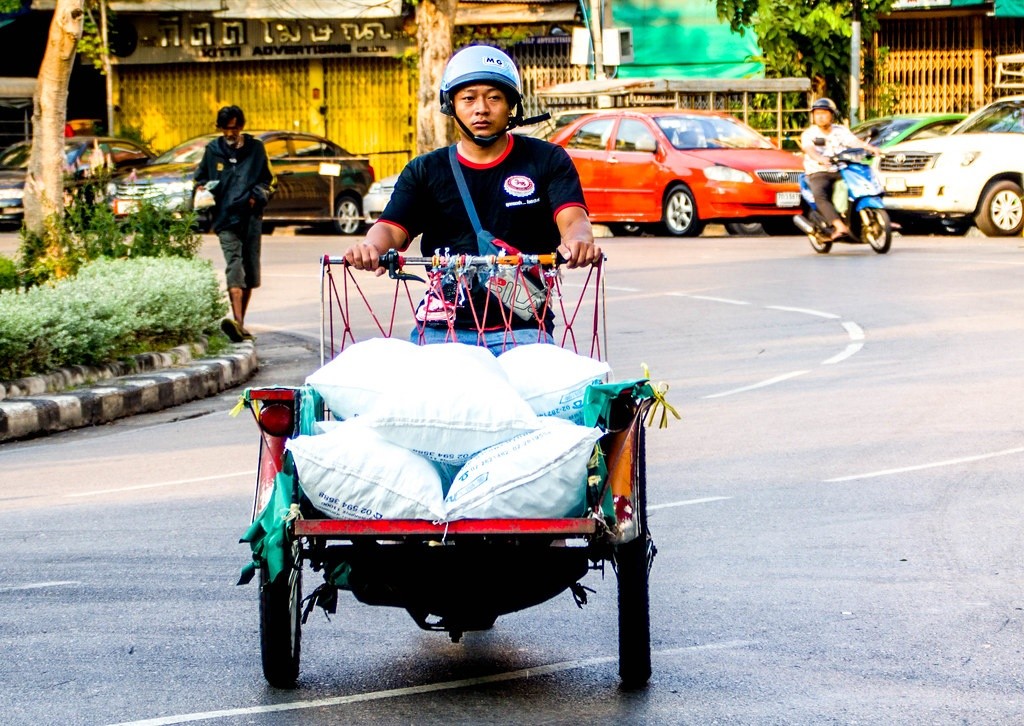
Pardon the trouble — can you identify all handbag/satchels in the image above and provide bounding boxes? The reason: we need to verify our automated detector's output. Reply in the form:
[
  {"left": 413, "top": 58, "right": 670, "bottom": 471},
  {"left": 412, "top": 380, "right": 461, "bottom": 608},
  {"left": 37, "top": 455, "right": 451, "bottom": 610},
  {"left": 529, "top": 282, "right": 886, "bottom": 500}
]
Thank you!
[{"left": 477, "top": 231, "right": 546, "bottom": 321}]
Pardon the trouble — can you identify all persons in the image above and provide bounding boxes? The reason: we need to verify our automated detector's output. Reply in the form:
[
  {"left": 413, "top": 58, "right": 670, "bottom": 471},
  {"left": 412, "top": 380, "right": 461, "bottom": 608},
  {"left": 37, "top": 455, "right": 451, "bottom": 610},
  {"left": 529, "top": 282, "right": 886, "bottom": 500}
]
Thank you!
[
  {"left": 192, "top": 104, "right": 273, "bottom": 343},
  {"left": 344, "top": 43, "right": 601, "bottom": 357},
  {"left": 800, "top": 97, "right": 901, "bottom": 241}
]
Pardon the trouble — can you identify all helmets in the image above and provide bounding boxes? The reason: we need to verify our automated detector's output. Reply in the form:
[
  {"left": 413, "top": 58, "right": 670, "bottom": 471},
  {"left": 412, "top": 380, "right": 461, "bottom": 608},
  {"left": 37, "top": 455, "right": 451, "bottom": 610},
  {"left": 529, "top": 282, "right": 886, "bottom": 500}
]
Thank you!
[
  {"left": 440, "top": 45, "right": 526, "bottom": 100},
  {"left": 812, "top": 98, "right": 842, "bottom": 120}
]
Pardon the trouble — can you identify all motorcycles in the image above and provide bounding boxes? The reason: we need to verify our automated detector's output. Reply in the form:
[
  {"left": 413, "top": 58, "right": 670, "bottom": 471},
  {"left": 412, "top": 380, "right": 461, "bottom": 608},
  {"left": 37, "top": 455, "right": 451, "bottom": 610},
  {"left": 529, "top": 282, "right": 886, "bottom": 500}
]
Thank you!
[{"left": 793, "top": 128, "right": 891, "bottom": 253}]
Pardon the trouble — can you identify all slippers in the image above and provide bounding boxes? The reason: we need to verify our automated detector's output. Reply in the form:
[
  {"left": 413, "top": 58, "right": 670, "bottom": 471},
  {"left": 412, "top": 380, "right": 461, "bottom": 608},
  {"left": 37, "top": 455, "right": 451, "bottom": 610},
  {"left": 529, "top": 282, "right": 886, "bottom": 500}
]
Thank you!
[{"left": 833, "top": 231, "right": 856, "bottom": 243}]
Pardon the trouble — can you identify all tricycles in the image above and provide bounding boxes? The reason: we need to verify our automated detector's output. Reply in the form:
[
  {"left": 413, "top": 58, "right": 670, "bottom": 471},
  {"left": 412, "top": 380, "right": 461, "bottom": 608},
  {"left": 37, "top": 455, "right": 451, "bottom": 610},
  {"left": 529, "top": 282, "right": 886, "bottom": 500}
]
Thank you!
[{"left": 242, "top": 254, "right": 657, "bottom": 690}]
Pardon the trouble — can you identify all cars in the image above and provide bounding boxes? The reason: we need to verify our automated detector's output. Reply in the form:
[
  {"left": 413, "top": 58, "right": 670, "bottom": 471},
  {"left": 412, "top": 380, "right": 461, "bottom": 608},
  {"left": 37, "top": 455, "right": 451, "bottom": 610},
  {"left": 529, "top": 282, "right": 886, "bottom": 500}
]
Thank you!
[
  {"left": 363, "top": 122, "right": 535, "bottom": 224},
  {"left": 102, "top": 130, "right": 376, "bottom": 236},
  {"left": 880, "top": 94, "right": 1024, "bottom": 237},
  {"left": 0, "top": 135, "right": 158, "bottom": 229},
  {"left": 850, "top": 112, "right": 966, "bottom": 234},
  {"left": 550, "top": 109, "right": 803, "bottom": 237}
]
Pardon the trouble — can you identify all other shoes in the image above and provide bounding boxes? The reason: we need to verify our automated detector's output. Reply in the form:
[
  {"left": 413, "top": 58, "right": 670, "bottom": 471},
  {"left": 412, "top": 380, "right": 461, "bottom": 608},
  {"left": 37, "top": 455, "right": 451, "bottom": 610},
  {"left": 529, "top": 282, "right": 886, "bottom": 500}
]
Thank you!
[{"left": 221, "top": 319, "right": 252, "bottom": 342}]
